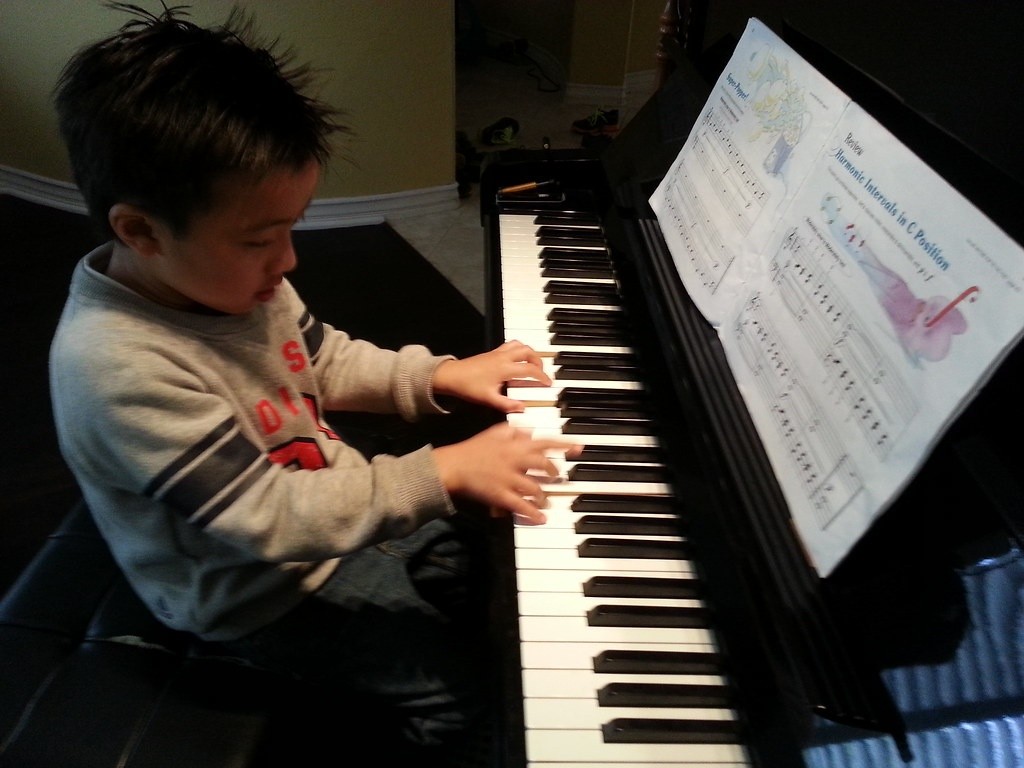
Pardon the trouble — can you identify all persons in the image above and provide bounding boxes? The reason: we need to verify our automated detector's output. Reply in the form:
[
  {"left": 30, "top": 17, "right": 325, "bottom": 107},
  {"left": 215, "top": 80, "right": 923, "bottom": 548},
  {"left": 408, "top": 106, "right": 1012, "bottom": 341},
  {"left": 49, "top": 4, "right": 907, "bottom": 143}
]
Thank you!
[{"left": 46, "top": 0, "right": 586, "bottom": 768}]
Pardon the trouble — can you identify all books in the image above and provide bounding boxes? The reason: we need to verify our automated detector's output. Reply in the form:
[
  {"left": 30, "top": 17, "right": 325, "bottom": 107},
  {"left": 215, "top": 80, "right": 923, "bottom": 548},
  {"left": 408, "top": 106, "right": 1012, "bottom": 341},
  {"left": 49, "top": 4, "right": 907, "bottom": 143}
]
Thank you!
[{"left": 646, "top": 17, "right": 1024, "bottom": 586}]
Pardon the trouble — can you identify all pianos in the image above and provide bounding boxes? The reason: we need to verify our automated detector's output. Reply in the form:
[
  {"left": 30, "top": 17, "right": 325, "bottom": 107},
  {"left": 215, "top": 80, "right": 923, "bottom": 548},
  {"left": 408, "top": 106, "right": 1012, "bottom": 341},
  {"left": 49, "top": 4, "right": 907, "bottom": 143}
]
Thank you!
[{"left": 477, "top": 0, "right": 1024, "bottom": 768}]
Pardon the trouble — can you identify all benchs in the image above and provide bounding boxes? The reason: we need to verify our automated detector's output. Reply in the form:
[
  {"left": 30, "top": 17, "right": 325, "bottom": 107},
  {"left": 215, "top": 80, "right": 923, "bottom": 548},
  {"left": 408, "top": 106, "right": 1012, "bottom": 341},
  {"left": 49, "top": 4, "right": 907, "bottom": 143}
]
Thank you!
[{"left": 0, "top": 490, "right": 274, "bottom": 768}]
